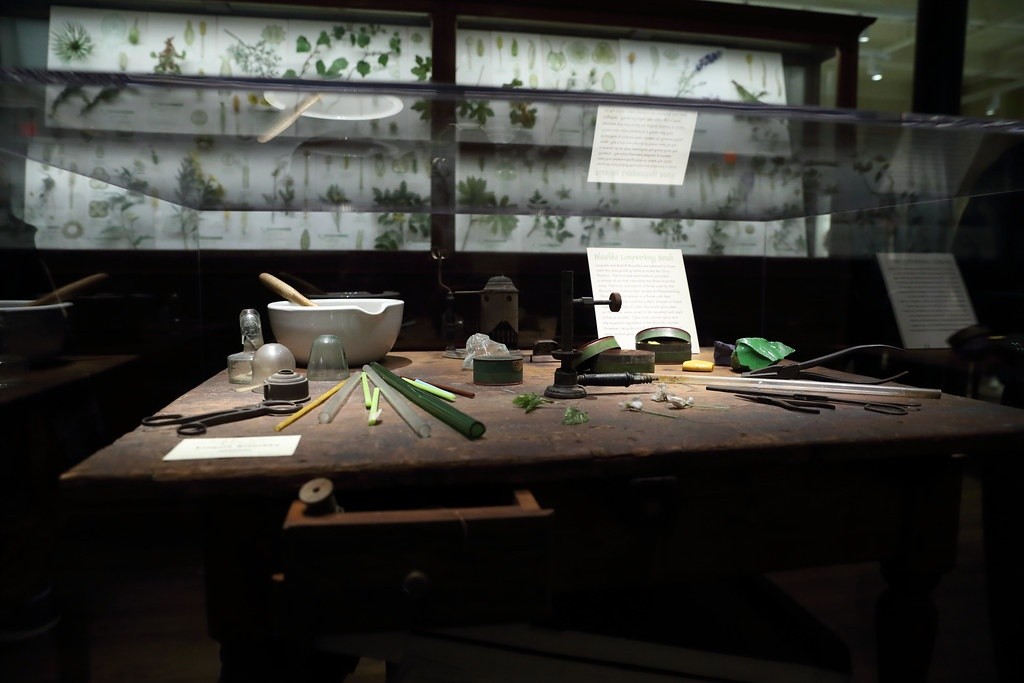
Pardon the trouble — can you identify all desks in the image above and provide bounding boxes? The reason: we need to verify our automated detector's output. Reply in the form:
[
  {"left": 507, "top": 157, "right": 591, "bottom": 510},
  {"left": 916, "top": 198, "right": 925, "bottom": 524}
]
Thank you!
[{"left": 57, "top": 346, "right": 1022, "bottom": 682}]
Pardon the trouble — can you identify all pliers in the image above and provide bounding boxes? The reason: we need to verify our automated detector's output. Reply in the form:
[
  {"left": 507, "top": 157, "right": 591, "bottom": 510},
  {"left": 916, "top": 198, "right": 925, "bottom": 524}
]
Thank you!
[{"left": 732, "top": 392, "right": 835, "bottom": 417}]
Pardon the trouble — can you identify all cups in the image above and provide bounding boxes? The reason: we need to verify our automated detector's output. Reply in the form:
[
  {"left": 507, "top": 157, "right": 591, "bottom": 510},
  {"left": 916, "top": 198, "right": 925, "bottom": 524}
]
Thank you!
[{"left": 304, "top": 333, "right": 351, "bottom": 380}]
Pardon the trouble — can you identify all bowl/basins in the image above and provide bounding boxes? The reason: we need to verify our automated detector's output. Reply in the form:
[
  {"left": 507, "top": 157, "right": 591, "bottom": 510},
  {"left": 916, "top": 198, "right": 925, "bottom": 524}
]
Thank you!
[{"left": 267, "top": 297, "right": 405, "bottom": 367}]
